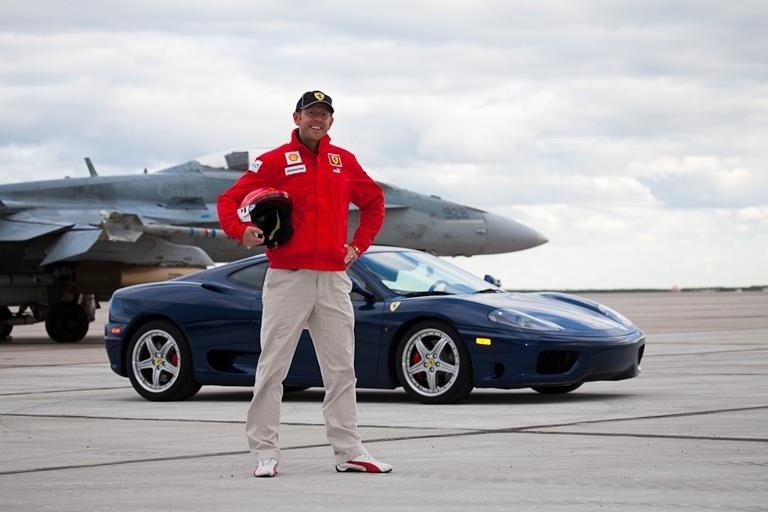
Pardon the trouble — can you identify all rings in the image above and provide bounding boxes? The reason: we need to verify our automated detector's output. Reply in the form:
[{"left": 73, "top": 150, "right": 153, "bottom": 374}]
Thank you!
[{"left": 346, "top": 255, "right": 354, "bottom": 258}]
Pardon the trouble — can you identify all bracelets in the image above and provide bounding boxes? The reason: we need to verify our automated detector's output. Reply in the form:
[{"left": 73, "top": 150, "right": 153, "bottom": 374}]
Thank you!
[{"left": 354, "top": 247, "right": 359, "bottom": 254}]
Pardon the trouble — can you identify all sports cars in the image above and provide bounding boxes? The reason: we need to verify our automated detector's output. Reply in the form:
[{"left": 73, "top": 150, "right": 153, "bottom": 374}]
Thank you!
[{"left": 99, "top": 239, "right": 653, "bottom": 406}]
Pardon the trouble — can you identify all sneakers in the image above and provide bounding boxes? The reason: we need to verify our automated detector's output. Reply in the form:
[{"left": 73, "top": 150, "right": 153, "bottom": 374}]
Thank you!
[
  {"left": 253, "top": 457, "right": 279, "bottom": 478},
  {"left": 334, "top": 453, "right": 393, "bottom": 473}
]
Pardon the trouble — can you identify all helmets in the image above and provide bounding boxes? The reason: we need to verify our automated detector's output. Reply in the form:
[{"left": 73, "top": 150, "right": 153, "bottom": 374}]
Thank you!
[{"left": 236, "top": 187, "right": 295, "bottom": 250}]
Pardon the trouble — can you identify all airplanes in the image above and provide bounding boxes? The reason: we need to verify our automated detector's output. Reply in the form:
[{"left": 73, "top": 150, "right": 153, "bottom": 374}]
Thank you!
[{"left": 1, "top": 148, "right": 551, "bottom": 347}]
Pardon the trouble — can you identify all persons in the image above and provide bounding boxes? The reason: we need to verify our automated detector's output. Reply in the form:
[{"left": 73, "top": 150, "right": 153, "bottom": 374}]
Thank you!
[{"left": 217, "top": 91, "right": 392, "bottom": 477}]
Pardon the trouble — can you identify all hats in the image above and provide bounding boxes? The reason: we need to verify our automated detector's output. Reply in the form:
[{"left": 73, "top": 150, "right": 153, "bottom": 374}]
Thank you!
[{"left": 295, "top": 90, "right": 335, "bottom": 114}]
